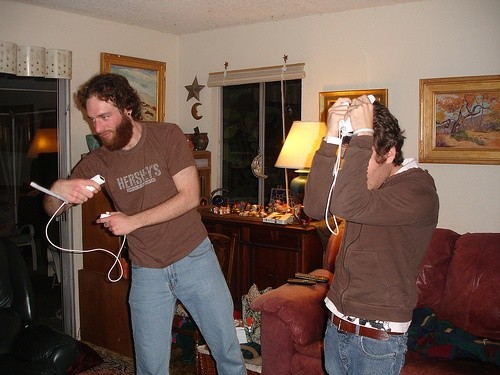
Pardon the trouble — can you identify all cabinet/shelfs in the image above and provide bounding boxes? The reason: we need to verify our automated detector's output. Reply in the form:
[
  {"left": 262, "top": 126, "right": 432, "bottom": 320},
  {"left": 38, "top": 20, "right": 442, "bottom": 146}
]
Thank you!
[{"left": 78, "top": 151, "right": 211, "bottom": 358}]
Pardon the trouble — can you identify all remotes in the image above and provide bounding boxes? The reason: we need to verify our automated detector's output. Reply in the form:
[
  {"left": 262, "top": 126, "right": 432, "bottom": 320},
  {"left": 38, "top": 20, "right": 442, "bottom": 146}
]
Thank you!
[
  {"left": 295, "top": 272, "right": 329, "bottom": 282},
  {"left": 288, "top": 278, "right": 316, "bottom": 285}
]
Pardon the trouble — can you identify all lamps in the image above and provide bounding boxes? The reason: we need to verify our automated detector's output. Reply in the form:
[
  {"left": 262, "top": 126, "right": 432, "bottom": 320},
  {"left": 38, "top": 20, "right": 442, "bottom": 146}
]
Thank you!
[
  {"left": 274, "top": 121, "right": 327, "bottom": 202},
  {"left": 27, "top": 127, "right": 57, "bottom": 158}
]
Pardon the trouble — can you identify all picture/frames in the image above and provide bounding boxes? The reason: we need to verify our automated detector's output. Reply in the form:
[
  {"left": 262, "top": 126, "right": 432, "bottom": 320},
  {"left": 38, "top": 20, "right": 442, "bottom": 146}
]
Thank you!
[
  {"left": 418, "top": 74, "right": 500, "bottom": 165},
  {"left": 318, "top": 88, "right": 389, "bottom": 126},
  {"left": 100, "top": 52, "right": 166, "bottom": 122}
]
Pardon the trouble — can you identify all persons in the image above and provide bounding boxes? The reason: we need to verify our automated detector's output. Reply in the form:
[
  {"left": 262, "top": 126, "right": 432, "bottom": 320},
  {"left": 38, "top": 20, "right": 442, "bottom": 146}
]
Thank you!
[
  {"left": 44, "top": 72, "right": 248, "bottom": 375},
  {"left": 303, "top": 95, "right": 440, "bottom": 375}
]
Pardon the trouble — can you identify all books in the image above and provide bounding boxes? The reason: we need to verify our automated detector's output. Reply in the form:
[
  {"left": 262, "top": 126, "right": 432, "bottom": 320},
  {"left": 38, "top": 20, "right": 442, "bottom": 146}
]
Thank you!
[{"left": 262, "top": 212, "right": 293, "bottom": 225}]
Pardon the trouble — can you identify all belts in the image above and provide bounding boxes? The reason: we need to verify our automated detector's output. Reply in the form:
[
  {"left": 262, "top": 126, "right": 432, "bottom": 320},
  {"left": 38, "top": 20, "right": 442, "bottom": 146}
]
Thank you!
[{"left": 327, "top": 311, "right": 404, "bottom": 341}]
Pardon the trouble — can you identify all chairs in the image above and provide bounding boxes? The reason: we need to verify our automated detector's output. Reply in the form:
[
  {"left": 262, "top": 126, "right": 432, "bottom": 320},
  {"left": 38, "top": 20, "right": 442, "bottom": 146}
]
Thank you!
[
  {"left": 172, "top": 231, "right": 237, "bottom": 375},
  {"left": 12, "top": 225, "right": 37, "bottom": 270}
]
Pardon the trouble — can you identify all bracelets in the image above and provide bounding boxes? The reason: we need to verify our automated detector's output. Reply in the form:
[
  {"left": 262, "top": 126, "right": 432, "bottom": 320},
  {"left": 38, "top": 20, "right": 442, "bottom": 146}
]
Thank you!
[{"left": 353, "top": 128, "right": 375, "bottom": 136}]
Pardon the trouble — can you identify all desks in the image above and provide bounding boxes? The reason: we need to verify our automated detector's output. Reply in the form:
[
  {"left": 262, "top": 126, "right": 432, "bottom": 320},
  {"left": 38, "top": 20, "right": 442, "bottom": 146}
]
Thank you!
[{"left": 197, "top": 206, "right": 338, "bottom": 312}]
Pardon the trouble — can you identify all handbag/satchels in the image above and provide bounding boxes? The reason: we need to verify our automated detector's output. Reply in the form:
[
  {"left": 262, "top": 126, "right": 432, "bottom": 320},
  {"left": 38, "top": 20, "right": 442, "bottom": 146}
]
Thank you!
[
  {"left": 414, "top": 316, "right": 499, "bottom": 365},
  {"left": 406, "top": 307, "right": 437, "bottom": 351}
]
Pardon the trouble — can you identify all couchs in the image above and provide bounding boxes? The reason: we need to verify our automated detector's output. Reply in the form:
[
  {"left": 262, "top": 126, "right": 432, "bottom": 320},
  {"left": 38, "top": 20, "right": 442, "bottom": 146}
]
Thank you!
[
  {"left": 249, "top": 230, "right": 500, "bottom": 375},
  {"left": 0, "top": 306, "right": 103, "bottom": 375}
]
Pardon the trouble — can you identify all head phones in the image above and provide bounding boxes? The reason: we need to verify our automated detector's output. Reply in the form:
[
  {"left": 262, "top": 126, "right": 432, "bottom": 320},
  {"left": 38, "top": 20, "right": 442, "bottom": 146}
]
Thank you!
[{"left": 209, "top": 188, "right": 234, "bottom": 207}]
